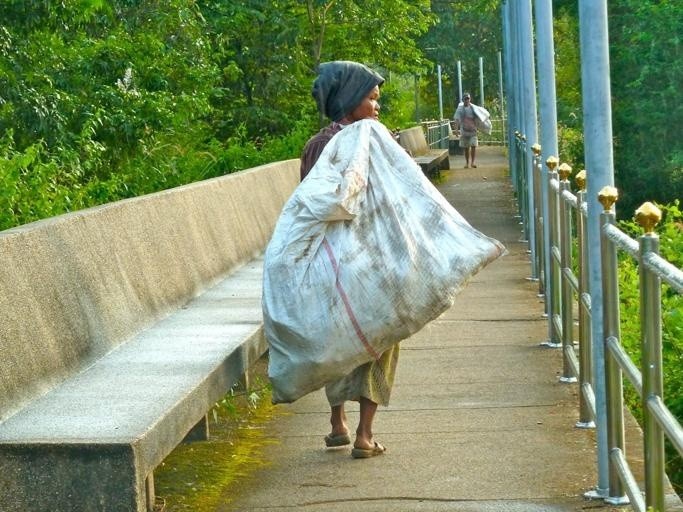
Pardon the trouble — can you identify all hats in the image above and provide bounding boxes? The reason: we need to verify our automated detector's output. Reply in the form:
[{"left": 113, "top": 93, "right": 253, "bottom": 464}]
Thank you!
[{"left": 462, "top": 93, "right": 470, "bottom": 99}]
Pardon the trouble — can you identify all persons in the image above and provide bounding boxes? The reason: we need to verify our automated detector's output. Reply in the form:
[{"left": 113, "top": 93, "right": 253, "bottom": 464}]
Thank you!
[
  {"left": 454, "top": 92, "right": 480, "bottom": 169},
  {"left": 299, "top": 60, "right": 399, "bottom": 459}
]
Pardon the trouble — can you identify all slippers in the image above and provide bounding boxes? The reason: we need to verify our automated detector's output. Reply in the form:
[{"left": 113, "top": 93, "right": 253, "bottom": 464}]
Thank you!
[
  {"left": 324, "top": 433, "right": 351, "bottom": 447},
  {"left": 352, "top": 441, "right": 387, "bottom": 458}
]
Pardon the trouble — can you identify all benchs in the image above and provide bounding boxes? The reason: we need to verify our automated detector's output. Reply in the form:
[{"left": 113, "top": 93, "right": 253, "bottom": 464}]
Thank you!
[
  {"left": 398, "top": 126, "right": 451, "bottom": 178},
  {"left": 0, "top": 156, "right": 302, "bottom": 512}
]
